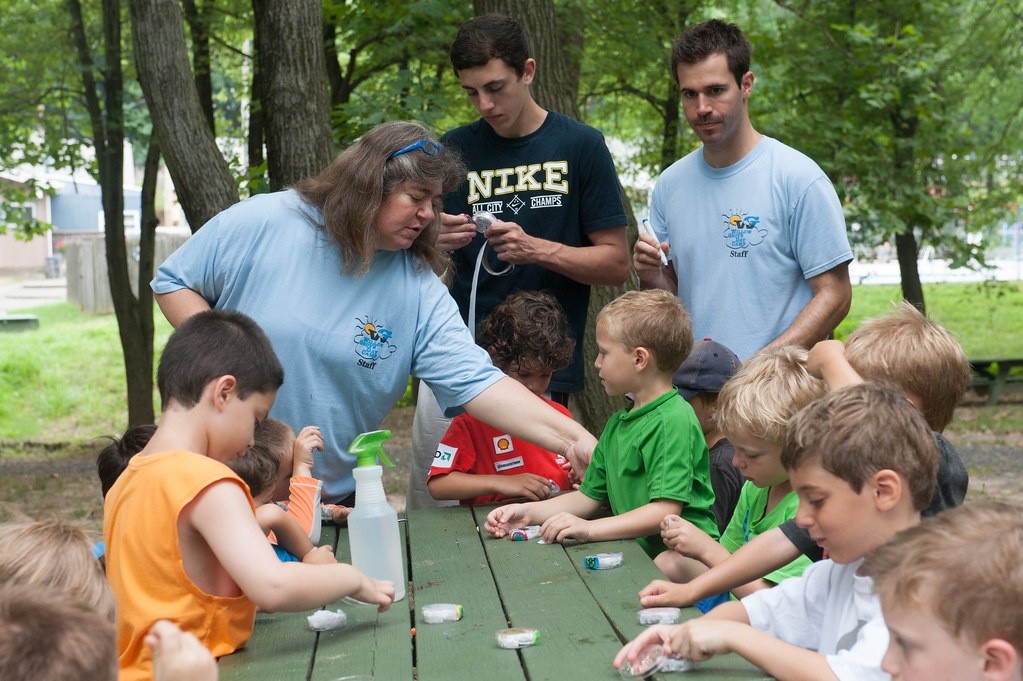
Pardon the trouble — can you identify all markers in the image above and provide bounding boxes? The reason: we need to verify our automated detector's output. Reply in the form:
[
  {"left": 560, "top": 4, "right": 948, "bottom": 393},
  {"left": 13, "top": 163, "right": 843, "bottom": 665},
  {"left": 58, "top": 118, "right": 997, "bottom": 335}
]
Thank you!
[{"left": 643, "top": 216, "right": 669, "bottom": 269}]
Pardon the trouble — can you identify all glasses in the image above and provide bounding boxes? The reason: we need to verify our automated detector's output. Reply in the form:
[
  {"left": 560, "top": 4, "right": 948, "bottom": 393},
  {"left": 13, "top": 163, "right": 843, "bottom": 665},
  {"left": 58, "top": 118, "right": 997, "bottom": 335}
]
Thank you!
[{"left": 388, "top": 140, "right": 442, "bottom": 160}]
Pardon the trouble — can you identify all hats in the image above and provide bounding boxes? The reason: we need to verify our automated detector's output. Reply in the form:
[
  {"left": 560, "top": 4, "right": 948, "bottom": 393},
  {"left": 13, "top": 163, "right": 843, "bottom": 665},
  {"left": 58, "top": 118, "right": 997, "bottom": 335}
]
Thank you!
[{"left": 672, "top": 337, "right": 745, "bottom": 401}]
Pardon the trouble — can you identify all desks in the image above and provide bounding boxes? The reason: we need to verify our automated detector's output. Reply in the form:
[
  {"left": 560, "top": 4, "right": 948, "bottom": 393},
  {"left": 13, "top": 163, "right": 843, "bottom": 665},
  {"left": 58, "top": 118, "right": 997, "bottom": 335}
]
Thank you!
[{"left": 217, "top": 504, "right": 776, "bottom": 681}]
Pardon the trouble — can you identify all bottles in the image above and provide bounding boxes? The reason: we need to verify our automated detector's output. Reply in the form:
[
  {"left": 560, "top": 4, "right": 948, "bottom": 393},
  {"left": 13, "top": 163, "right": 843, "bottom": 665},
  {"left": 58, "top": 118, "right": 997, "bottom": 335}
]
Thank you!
[{"left": 346, "top": 429, "right": 406, "bottom": 605}]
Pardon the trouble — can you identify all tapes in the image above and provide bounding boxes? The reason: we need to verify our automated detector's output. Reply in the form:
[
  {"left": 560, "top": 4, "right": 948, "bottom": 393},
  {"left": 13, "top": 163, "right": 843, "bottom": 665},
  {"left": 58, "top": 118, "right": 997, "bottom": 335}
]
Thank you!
[{"left": 479, "top": 240, "right": 515, "bottom": 276}]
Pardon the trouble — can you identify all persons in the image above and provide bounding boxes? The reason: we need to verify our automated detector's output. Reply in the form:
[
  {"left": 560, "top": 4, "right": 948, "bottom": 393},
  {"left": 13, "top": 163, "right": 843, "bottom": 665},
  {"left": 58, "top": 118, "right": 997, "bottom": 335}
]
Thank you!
[
  {"left": 0, "top": 511, "right": 221, "bottom": 681},
  {"left": 613, "top": 379, "right": 940, "bottom": 681},
  {"left": 95, "top": 286, "right": 970, "bottom": 681},
  {"left": 632, "top": 18, "right": 854, "bottom": 363},
  {"left": 858, "top": 497, "right": 1023, "bottom": 681},
  {"left": 405, "top": 12, "right": 632, "bottom": 506},
  {"left": 144, "top": 118, "right": 600, "bottom": 508}
]
